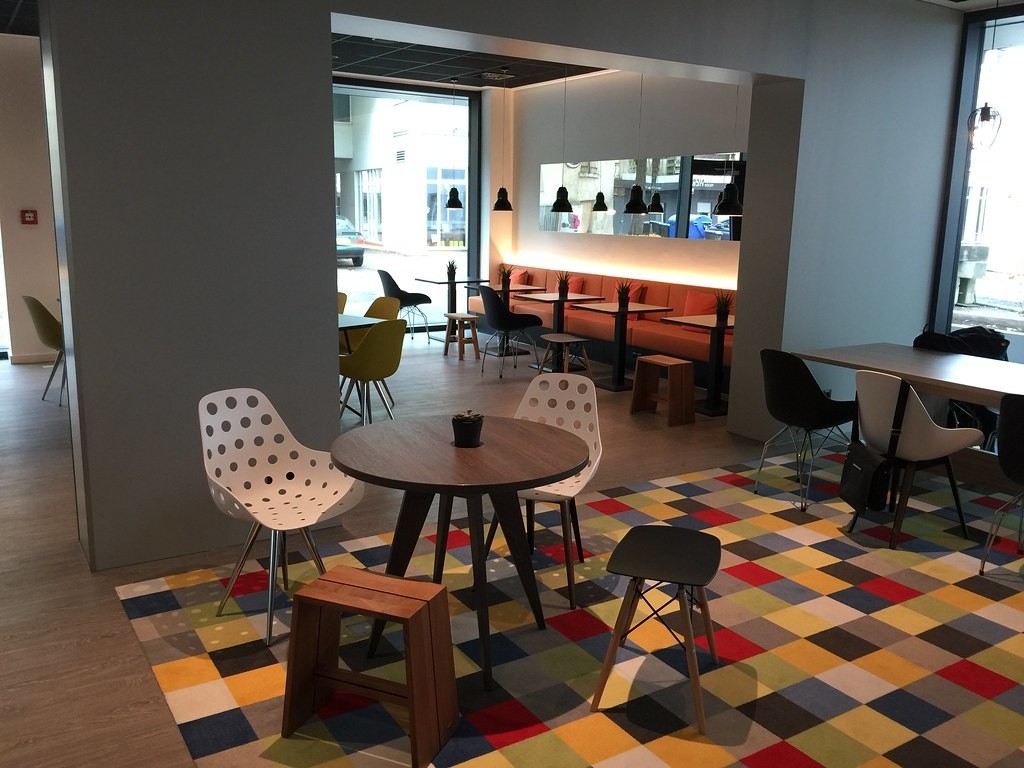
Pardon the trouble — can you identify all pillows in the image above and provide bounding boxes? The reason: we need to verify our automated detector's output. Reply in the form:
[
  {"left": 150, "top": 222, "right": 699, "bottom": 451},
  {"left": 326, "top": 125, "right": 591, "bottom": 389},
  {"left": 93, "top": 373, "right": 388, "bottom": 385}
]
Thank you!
[{"left": 508, "top": 270, "right": 718, "bottom": 333}]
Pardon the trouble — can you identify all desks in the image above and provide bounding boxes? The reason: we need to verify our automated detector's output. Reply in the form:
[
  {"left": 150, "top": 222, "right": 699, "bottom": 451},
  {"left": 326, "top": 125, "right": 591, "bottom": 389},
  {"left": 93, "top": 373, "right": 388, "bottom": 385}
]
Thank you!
[
  {"left": 330, "top": 277, "right": 737, "bottom": 691},
  {"left": 338, "top": 314, "right": 389, "bottom": 425},
  {"left": 791, "top": 342, "right": 1024, "bottom": 500}
]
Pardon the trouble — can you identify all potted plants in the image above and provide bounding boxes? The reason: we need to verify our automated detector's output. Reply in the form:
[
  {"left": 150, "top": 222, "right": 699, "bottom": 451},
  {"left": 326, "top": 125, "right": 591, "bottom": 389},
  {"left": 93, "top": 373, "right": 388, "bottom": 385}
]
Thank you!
[
  {"left": 452, "top": 409, "right": 484, "bottom": 448},
  {"left": 445, "top": 262, "right": 733, "bottom": 320}
]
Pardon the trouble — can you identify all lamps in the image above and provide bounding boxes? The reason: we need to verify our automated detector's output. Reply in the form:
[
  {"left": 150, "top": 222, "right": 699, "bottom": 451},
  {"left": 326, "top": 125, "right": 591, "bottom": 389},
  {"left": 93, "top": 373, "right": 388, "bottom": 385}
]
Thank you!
[
  {"left": 445, "top": 62, "right": 744, "bottom": 216},
  {"left": 969, "top": 1, "right": 1002, "bottom": 149}
]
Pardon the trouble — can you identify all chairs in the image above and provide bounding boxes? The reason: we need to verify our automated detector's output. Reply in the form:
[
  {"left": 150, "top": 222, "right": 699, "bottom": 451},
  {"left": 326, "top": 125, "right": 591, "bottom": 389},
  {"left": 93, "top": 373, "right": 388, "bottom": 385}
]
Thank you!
[
  {"left": 21, "top": 296, "right": 67, "bottom": 406},
  {"left": 198, "top": 270, "right": 1024, "bottom": 647}
]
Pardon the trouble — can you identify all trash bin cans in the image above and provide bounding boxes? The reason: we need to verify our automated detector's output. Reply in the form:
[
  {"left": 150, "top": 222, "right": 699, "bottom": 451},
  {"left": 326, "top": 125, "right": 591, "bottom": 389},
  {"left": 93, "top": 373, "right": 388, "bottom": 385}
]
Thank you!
[
  {"left": 703, "top": 226, "right": 730, "bottom": 240},
  {"left": 667, "top": 214, "right": 712, "bottom": 238}
]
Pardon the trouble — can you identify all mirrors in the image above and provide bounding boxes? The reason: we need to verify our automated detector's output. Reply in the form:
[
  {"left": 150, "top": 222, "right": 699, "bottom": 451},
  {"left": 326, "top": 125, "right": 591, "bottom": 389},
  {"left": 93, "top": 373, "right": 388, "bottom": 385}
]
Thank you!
[
  {"left": 539, "top": 151, "right": 746, "bottom": 242},
  {"left": 712, "top": 153, "right": 728, "bottom": 214},
  {"left": 592, "top": 161, "right": 608, "bottom": 211},
  {"left": 647, "top": 157, "right": 663, "bottom": 214}
]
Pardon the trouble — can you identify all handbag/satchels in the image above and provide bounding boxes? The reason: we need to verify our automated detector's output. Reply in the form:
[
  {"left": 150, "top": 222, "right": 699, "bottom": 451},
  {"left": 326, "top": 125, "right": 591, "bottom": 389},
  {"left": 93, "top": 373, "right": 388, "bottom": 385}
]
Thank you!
[{"left": 837, "top": 441, "right": 894, "bottom": 512}]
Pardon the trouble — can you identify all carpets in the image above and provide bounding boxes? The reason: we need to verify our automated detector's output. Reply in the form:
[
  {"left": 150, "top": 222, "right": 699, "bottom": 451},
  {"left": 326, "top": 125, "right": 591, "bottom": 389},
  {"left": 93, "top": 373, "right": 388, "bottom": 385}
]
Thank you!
[{"left": 115, "top": 443, "right": 1024, "bottom": 768}]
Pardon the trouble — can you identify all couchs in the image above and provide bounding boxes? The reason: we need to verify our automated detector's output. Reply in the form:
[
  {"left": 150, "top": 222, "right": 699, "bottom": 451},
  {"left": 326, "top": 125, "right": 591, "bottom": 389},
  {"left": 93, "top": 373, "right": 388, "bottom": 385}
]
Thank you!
[{"left": 468, "top": 263, "right": 741, "bottom": 396}]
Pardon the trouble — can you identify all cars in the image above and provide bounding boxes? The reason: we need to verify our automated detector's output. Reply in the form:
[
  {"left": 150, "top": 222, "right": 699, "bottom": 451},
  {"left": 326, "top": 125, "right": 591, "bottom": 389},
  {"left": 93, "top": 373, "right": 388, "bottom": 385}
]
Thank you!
[{"left": 336, "top": 215, "right": 364, "bottom": 266}]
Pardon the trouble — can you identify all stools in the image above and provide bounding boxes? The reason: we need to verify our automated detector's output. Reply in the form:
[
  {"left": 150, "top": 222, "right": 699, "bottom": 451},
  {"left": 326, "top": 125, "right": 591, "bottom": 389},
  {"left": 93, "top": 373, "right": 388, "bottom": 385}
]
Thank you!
[
  {"left": 282, "top": 526, "right": 720, "bottom": 768},
  {"left": 444, "top": 314, "right": 481, "bottom": 360},
  {"left": 539, "top": 333, "right": 596, "bottom": 387},
  {"left": 630, "top": 355, "right": 696, "bottom": 426}
]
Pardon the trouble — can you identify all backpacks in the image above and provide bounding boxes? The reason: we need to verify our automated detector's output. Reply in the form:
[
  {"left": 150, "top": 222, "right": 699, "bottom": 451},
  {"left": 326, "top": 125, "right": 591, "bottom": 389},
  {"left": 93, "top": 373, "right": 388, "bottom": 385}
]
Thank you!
[{"left": 913, "top": 323, "right": 1010, "bottom": 357}]
